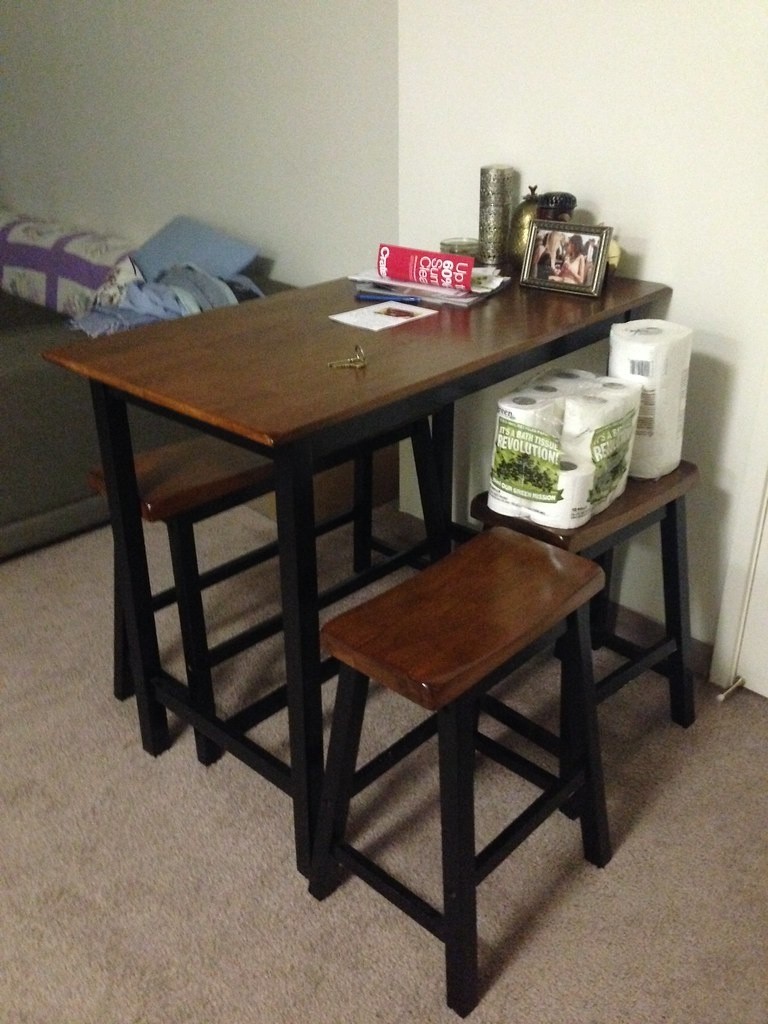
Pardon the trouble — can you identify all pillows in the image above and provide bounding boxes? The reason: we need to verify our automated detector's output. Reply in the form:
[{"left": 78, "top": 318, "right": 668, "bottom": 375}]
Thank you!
[{"left": 0, "top": 208, "right": 139, "bottom": 315}]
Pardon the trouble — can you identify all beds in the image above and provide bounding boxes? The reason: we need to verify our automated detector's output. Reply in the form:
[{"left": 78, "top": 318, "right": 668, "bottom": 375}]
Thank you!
[{"left": 1, "top": 274, "right": 308, "bottom": 565}]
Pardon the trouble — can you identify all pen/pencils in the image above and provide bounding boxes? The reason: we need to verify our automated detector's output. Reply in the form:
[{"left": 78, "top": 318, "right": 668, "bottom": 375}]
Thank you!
[{"left": 354, "top": 294, "right": 422, "bottom": 302}]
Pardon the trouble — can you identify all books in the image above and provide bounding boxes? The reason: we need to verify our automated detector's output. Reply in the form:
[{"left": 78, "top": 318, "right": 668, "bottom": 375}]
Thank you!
[{"left": 356, "top": 276, "right": 511, "bottom": 307}]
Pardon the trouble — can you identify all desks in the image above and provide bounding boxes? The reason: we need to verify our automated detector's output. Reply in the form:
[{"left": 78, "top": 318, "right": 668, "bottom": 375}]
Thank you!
[{"left": 40, "top": 275, "right": 667, "bottom": 874}]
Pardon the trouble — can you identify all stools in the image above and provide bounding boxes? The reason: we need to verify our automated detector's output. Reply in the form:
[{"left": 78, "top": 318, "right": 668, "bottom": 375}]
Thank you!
[
  {"left": 464, "top": 455, "right": 700, "bottom": 820},
  {"left": 311, "top": 523, "right": 608, "bottom": 1016},
  {"left": 89, "top": 414, "right": 438, "bottom": 763}
]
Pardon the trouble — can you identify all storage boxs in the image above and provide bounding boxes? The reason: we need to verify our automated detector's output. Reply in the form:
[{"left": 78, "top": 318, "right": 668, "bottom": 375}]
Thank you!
[{"left": 244, "top": 440, "right": 402, "bottom": 529}]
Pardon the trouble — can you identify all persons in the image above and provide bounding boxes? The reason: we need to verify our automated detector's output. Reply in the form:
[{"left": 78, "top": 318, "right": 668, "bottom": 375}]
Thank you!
[{"left": 532, "top": 231, "right": 598, "bottom": 285}]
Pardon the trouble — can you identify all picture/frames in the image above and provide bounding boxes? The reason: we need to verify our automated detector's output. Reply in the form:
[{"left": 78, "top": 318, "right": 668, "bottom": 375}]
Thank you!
[{"left": 520, "top": 219, "right": 613, "bottom": 298}]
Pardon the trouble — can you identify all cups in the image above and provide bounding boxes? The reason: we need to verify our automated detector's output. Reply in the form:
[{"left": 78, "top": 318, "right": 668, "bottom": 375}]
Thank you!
[
  {"left": 479, "top": 166, "right": 516, "bottom": 265},
  {"left": 440, "top": 238, "right": 479, "bottom": 267}
]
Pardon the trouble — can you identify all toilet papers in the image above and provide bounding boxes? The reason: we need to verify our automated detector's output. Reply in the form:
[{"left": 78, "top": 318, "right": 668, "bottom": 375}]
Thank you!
[{"left": 487, "top": 316, "right": 697, "bottom": 531}]
